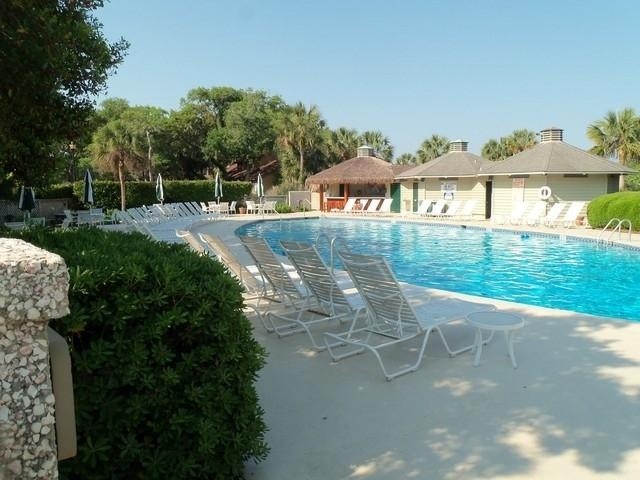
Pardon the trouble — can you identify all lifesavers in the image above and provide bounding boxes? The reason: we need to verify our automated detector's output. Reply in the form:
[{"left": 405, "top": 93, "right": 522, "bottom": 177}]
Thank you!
[{"left": 538, "top": 186, "right": 551, "bottom": 200}]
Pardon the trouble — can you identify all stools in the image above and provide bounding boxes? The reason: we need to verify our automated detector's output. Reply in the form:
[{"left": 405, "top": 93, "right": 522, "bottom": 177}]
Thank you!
[{"left": 468, "top": 308, "right": 526, "bottom": 370}]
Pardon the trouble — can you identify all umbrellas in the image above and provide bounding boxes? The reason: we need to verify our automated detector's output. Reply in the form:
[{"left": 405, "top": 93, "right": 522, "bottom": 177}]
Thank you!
[
  {"left": 215, "top": 172, "right": 224, "bottom": 204},
  {"left": 257, "top": 173, "right": 264, "bottom": 204},
  {"left": 84, "top": 169, "right": 95, "bottom": 214},
  {"left": 156, "top": 173, "right": 164, "bottom": 204},
  {"left": 18, "top": 185, "right": 36, "bottom": 223}
]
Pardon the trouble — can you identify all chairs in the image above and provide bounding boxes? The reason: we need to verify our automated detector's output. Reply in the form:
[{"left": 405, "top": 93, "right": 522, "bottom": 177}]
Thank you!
[
  {"left": 71, "top": 200, "right": 282, "bottom": 230},
  {"left": 174, "top": 229, "right": 355, "bottom": 335},
  {"left": 323, "top": 249, "right": 497, "bottom": 379},
  {"left": 268, "top": 240, "right": 429, "bottom": 351},
  {"left": 329, "top": 197, "right": 585, "bottom": 229}
]
